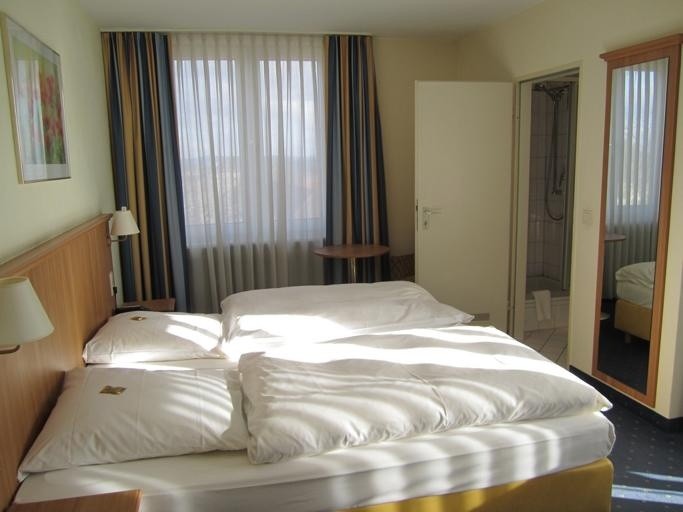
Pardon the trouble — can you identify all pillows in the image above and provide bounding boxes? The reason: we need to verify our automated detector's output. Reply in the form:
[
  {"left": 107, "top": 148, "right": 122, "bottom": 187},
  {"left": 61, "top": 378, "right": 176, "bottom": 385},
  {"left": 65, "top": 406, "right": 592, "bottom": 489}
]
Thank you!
[
  {"left": 16, "top": 365, "right": 249, "bottom": 483},
  {"left": 82, "top": 310, "right": 224, "bottom": 364}
]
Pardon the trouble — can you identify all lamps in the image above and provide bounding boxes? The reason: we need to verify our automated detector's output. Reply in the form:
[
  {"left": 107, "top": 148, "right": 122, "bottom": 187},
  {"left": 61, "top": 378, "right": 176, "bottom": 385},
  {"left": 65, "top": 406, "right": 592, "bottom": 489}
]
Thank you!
[
  {"left": 0, "top": 276, "right": 55, "bottom": 354},
  {"left": 107, "top": 209, "right": 141, "bottom": 248}
]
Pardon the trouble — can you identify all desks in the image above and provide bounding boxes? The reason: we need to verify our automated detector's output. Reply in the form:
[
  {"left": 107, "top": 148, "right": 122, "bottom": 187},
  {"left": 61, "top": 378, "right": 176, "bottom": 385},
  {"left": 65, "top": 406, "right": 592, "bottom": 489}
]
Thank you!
[
  {"left": 600, "top": 233, "right": 626, "bottom": 323},
  {"left": 312, "top": 244, "right": 390, "bottom": 283}
]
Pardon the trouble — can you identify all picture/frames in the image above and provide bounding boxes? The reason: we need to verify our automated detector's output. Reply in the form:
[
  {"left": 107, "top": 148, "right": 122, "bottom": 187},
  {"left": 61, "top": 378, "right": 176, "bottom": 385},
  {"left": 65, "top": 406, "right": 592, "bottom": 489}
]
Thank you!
[{"left": 1, "top": 10, "right": 71, "bottom": 185}]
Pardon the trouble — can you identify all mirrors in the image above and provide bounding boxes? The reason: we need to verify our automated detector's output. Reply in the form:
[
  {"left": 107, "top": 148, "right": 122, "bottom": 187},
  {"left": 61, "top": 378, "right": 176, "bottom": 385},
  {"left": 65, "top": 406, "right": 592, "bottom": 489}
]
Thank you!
[{"left": 592, "top": 32, "right": 683, "bottom": 408}]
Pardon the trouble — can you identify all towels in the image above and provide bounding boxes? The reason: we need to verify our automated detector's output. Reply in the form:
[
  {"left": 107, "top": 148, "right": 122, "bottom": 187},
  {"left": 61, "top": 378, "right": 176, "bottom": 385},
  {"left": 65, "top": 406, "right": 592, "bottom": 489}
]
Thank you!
[{"left": 531, "top": 290, "right": 551, "bottom": 321}]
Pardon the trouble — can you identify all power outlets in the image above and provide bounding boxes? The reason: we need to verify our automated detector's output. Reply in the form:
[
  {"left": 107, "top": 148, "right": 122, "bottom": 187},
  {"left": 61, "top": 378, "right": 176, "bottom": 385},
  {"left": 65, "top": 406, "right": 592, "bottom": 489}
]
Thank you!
[{"left": 109, "top": 271, "right": 116, "bottom": 297}]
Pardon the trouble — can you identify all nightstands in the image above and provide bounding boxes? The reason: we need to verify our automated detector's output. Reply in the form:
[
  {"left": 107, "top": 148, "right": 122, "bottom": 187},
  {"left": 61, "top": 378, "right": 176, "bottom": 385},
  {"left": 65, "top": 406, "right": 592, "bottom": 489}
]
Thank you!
[
  {"left": 116, "top": 298, "right": 176, "bottom": 311},
  {"left": 2, "top": 488, "right": 144, "bottom": 512}
]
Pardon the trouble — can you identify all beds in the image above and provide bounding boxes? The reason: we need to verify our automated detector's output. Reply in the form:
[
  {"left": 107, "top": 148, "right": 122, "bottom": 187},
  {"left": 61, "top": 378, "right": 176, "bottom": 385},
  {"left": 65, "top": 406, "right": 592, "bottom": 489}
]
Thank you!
[
  {"left": 614, "top": 261, "right": 656, "bottom": 342},
  {"left": 0, "top": 213, "right": 616, "bottom": 512}
]
